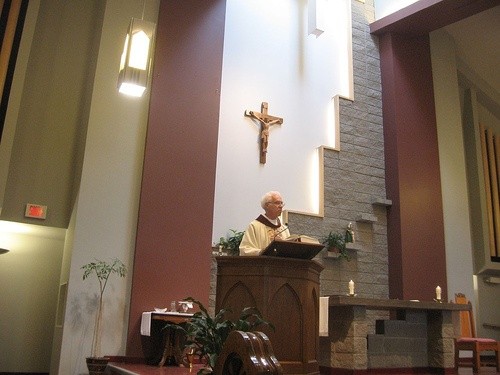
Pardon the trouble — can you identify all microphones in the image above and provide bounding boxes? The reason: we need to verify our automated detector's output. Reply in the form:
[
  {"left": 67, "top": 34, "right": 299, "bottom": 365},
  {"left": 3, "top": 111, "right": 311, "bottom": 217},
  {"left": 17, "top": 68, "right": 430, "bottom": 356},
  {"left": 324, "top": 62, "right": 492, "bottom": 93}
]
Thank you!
[{"left": 274, "top": 222, "right": 289, "bottom": 240}]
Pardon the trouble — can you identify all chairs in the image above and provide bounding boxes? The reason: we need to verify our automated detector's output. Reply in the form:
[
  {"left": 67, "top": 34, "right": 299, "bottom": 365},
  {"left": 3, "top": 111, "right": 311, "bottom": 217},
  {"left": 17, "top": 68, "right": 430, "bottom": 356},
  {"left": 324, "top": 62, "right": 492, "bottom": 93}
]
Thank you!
[{"left": 449, "top": 293, "right": 500, "bottom": 375}]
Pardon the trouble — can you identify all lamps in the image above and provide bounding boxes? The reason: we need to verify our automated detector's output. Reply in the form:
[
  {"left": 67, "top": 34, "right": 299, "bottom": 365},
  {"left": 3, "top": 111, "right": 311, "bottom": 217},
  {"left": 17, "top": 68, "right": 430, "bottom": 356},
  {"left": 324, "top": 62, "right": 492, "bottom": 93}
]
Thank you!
[
  {"left": 436, "top": 285, "right": 442, "bottom": 300},
  {"left": 117, "top": 0, "right": 156, "bottom": 98},
  {"left": 349, "top": 280, "right": 354, "bottom": 295}
]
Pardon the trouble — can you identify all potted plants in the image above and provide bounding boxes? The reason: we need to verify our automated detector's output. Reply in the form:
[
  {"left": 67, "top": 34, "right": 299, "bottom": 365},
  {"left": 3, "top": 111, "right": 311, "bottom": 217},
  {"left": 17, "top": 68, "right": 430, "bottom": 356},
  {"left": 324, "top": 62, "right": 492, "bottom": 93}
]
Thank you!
[
  {"left": 80, "top": 256, "right": 128, "bottom": 375},
  {"left": 324, "top": 233, "right": 350, "bottom": 261}
]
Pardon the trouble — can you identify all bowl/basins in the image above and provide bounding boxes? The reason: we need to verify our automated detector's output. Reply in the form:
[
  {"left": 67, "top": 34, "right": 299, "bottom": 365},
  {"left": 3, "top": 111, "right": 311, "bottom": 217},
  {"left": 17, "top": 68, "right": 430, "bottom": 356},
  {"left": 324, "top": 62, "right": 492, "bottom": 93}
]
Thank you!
[{"left": 155, "top": 308, "right": 167, "bottom": 313}]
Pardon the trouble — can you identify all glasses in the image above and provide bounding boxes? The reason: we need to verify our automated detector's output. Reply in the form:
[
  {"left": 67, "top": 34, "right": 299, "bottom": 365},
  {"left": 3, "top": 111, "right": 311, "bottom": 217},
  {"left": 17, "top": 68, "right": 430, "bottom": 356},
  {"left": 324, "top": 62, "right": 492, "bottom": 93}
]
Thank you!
[{"left": 268, "top": 201, "right": 285, "bottom": 207}]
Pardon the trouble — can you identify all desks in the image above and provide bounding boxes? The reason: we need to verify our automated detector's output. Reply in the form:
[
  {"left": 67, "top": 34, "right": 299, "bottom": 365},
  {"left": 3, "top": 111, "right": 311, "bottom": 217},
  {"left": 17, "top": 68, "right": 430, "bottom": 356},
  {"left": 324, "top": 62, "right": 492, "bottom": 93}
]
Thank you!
[
  {"left": 141, "top": 311, "right": 195, "bottom": 367},
  {"left": 324, "top": 294, "right": 472, "bottom": 369}
]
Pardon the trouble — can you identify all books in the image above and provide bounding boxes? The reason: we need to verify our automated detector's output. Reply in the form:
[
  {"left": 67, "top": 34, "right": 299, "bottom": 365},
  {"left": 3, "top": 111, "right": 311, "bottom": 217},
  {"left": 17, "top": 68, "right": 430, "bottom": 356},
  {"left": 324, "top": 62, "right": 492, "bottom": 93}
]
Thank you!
[{"left": 284, "top": 234, "right": 319, "bottom": 243}]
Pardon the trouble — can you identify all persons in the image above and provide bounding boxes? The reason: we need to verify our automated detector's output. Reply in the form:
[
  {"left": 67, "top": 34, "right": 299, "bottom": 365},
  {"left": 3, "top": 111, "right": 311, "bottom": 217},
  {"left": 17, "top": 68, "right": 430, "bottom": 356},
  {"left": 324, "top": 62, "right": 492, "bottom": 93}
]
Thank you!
[
  {"left": 249, "top": 111, "right": 281, "bottom": 153},
  {"left": 345, "top": 222, "right": 355, "bottom": 243},
  {"left": 436, "top": 286, "right": 442, "bottom": 299},
  {"left": 239, "top": 191, "right": 290, "bottom": 256},
  {"left": 349, "top": 280, "right": 354, "bottom": 294}
]
